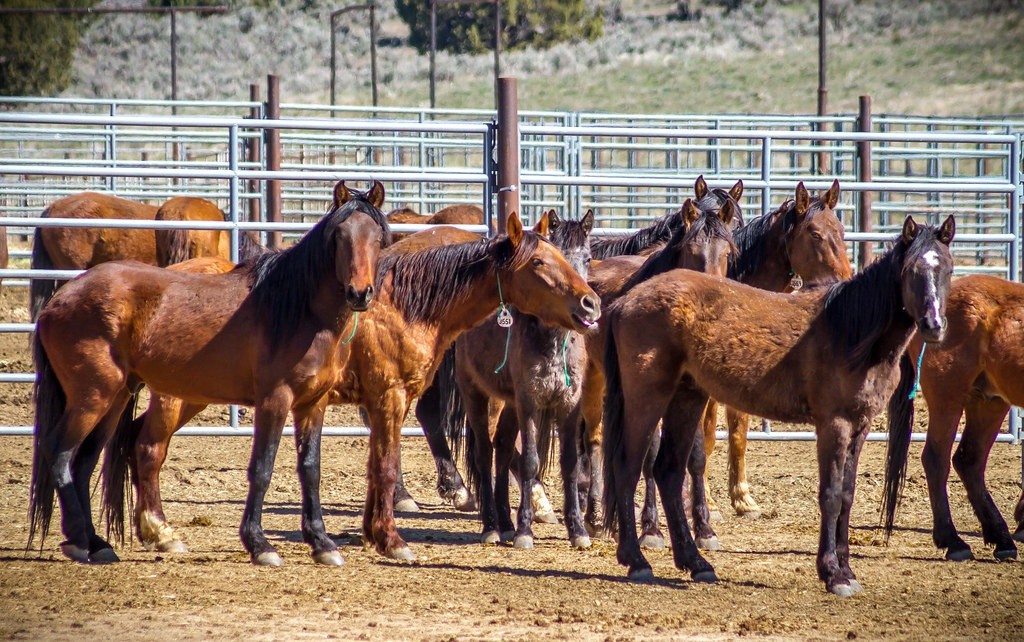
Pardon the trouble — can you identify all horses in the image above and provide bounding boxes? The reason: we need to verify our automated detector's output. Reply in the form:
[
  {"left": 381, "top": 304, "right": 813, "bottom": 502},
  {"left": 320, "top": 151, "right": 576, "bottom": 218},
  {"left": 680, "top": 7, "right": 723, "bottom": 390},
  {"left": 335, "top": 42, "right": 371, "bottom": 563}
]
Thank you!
[{"left": 21, "top": 174, "right": 1020, "bottom": 597}]
